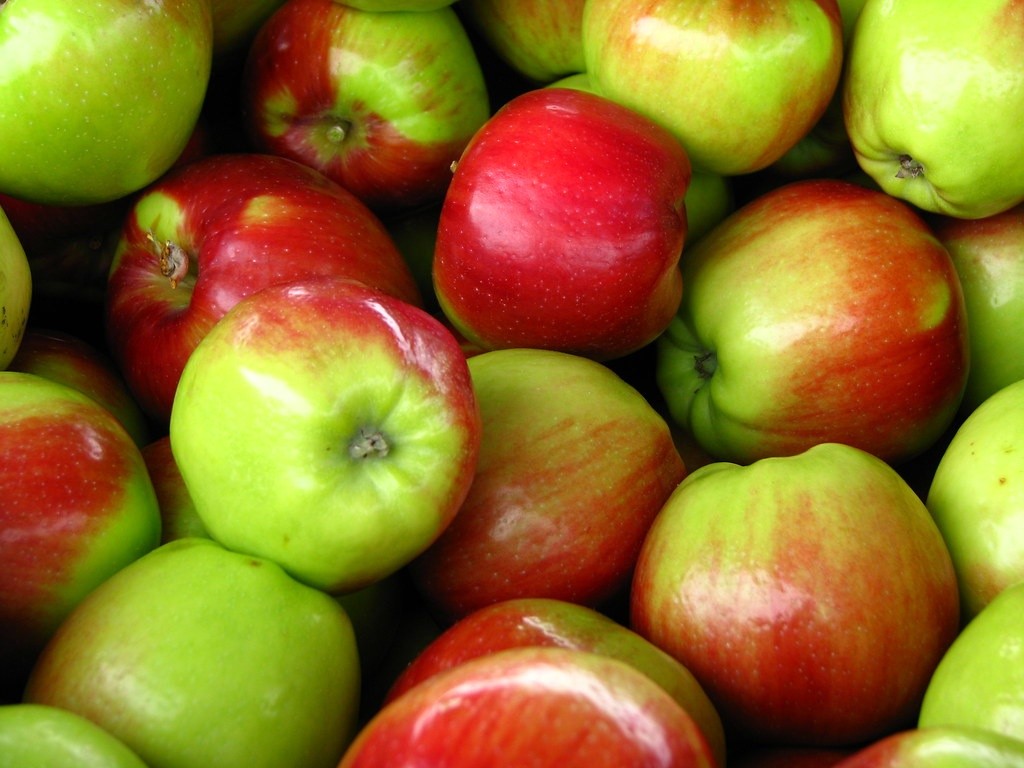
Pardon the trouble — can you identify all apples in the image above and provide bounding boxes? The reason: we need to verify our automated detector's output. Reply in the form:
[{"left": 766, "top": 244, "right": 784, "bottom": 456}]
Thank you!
[{"left": 0, "top": 0, "right": 1024, "bottom": 768}]
